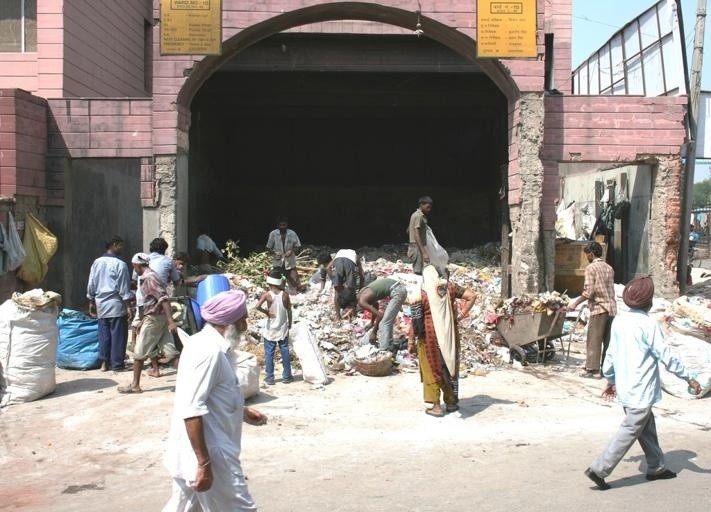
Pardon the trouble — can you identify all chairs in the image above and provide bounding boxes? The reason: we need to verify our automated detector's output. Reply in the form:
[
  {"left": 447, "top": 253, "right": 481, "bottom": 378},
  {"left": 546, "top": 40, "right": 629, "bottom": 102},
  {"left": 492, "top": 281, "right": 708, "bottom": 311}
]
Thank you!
[
  {"left": 585, "top": 468, "right": 610, "bottom": 489},
  {"left": 646, "top": 468, "right": 676, "bottom": 481}
]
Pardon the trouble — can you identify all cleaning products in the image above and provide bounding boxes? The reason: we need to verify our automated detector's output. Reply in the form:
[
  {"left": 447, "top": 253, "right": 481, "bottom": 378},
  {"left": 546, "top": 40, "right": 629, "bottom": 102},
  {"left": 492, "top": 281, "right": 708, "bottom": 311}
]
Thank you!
[
  {"left": 494, "top": 304, "right": 592, "bottom": 366},
  {"left": 163, "top": 269, "right": 198, "bottom": 336}
]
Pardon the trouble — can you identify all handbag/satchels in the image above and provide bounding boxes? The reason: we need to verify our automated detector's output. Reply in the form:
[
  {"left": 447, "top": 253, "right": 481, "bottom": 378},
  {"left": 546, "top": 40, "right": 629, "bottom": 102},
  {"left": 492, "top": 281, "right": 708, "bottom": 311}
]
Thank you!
[
  {"left": 456, "top": 312, "right": 464, "bottom": 321},
  {"left": 198, "top": 459, "right": 211, "bottom": 467}
]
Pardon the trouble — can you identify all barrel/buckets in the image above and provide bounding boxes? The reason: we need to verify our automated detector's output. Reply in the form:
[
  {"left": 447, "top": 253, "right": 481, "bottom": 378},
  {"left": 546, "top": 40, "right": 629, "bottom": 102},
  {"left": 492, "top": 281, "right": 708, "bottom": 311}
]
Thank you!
[
  {"left": 581, "top": 371, "right": 601, "bottom": 379},
  {"left": 426, "top": 409, "right": 444, "bottom": 417},
  {"left": 146, "top": 369, "right": 159, "bottom": 378},
  {"left": 447, "top": 405, "right": 460, "bottom": 411},
  {"left": 118, "top": 385, "right": 143, "bottom": 394}
]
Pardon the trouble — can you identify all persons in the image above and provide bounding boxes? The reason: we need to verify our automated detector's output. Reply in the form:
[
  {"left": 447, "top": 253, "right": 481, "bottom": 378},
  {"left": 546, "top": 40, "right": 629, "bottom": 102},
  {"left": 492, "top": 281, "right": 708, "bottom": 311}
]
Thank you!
[
  {"left": 585, "top": 276, "right": 702, "bottom": 490},
  {"left": 408, "top": 264, "right": 477, "bottom": 416},
  {"left": 315, "top": 248, "right": 408, "bottom": 354},
  {"left": 689, "top": 224, "right": 700, "bottom": 244},
  {"left": 406, "top": 196, "right": 433, "bottom": 275},
  {"left": 266, "top": 215, "right": 303, "bottom": 293},
  {"left": 256, "top": 270, "right": 292, "bottom": 385},
  {"left": 566, "top": 241, "right": 616, "bottom": 380},
  {"left": 676, "top": 235, "right": 697, "bottom": 286},
  {"left": 85, "top": 235, "right": 207, "bottom": 393},
  {"left": 161, "top": 290, "right": 267, "bottom": 512}
]
